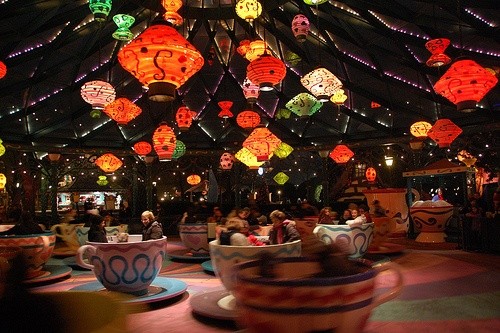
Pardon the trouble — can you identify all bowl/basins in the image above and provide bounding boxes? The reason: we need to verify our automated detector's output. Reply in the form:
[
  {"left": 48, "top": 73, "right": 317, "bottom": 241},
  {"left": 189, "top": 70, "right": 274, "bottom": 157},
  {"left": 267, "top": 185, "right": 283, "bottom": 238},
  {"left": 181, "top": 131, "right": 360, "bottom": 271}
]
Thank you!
[
  {"left": 179, "top": 222, "right": 224, "bottom": 255},
  {"left": 408, "top": 206, "right": 456, "bottom": 243},
  {"left": 208, "top": 235, "right": 302, "bottom": 299},
  {"left": 314, "top": 223, "right": 375, "bottom": 259},
  {"left": 0, "top": 230, "right": 56, "bottom": 278}
]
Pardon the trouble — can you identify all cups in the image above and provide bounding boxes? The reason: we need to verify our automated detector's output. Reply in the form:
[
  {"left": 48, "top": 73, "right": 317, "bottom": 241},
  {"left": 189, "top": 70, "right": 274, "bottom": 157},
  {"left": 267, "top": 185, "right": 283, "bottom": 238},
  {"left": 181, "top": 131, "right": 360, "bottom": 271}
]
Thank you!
[
  {"left": 292, "top": 217, "right": 319, "bottom": 245},
  {"left": 76, "top": 234, "right": 167, "bottom": 295},
  {"left": 105, "top": 225, "right": 128, "bottom": 243},
  {"left": 372, "top": 217, "right": 395, "bottom": 247},
  {"left": 233, "top": 257, "right": 404, "bottom": 333}
]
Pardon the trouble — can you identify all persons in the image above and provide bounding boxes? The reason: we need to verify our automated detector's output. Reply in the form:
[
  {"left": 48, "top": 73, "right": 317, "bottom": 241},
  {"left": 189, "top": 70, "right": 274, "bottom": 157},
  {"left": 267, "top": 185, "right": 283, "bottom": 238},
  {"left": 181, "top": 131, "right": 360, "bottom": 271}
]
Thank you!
[
  {"left": 243, "top": 206, "right": 267, "bottom": 224},
  {"left": 182, "top": 205, "right": 227, "bottom": 223},
  {"left": 226, "top": 209, "right": 247, "bottom": 220},
  {"left": 338, "top": 210, "right": 353, "bottom": 225},
  {"left": 433, "top": 189, "right": 446, "bottom": 203},
  {"left": 459, "top": 192, "right": 500, "bottom": 253},
  {"left": 349, "top": 203, "right": 372, "bottom": 223},
  {"left": 215, "top": 219, "right": 265, "bottom": 247},
  {"left": 141, "top": 211, "right": 163, "bottom": 241},
  {"left": 267, "top": 210, "right": 300, "bottom": 245},
  {"left": 88, "top": 215, "right": 107, "bottom": 245},
  {"left": 319, "top": 208, "right": 335, "bottom": 225},
  {"left": 372, "top": 200, "right": 390, "bottom": 217},
  {"left": 0, "top": 212, "right": 45, "bottom": 234},
  {"left": 346, "top": 209, "right": 365, "bottom": 227},
  {"left": 64, "top": 203, "right": 119, "bottom": 227}
]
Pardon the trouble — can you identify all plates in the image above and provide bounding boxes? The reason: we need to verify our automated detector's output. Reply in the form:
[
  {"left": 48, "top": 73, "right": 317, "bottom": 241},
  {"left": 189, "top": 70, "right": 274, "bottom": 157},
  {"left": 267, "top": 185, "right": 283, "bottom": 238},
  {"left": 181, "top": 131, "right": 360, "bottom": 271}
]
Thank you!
[
  {"left": 190, "top": 290, "right": 241, "bottom": 319},
  {"left": 168, "top": 248, "right": 211, "bottom": 259},
  {"left": 70, "top": 276, "right": 188, "bottom": 306},
  {"left": 0, "top": 262, "right": 72, "bottom": 284},
  {"left": 364, "top": 243, "right": 406, "bottom": 253}
]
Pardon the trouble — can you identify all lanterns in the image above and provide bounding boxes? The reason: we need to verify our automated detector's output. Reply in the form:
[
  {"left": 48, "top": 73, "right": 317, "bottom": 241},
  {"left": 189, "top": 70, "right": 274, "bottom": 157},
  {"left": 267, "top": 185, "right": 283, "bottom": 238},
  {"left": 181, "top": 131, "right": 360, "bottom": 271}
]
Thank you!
[
  {"left": 81, "top": 81, "right": 116, "bottom": 111},
  {"left": 90, "top": 0, "right": 466, "bottom": 186},
  {"left": 433, "top": 58, "right": 498, "bottom": 112},
  {"left": 117, "top": 23, "right": 207, "bottom": 101}
]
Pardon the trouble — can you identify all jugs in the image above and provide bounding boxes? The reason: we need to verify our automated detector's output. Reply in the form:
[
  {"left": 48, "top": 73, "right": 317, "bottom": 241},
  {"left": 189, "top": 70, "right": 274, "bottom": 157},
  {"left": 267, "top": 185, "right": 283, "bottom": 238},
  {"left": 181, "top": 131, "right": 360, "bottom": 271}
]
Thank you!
[
  {"left": 51, "top": 223, "right": 90, "bottom": 244},
  {"left": 362, "top": 188, "right": 420, "bottom": 241}
]
